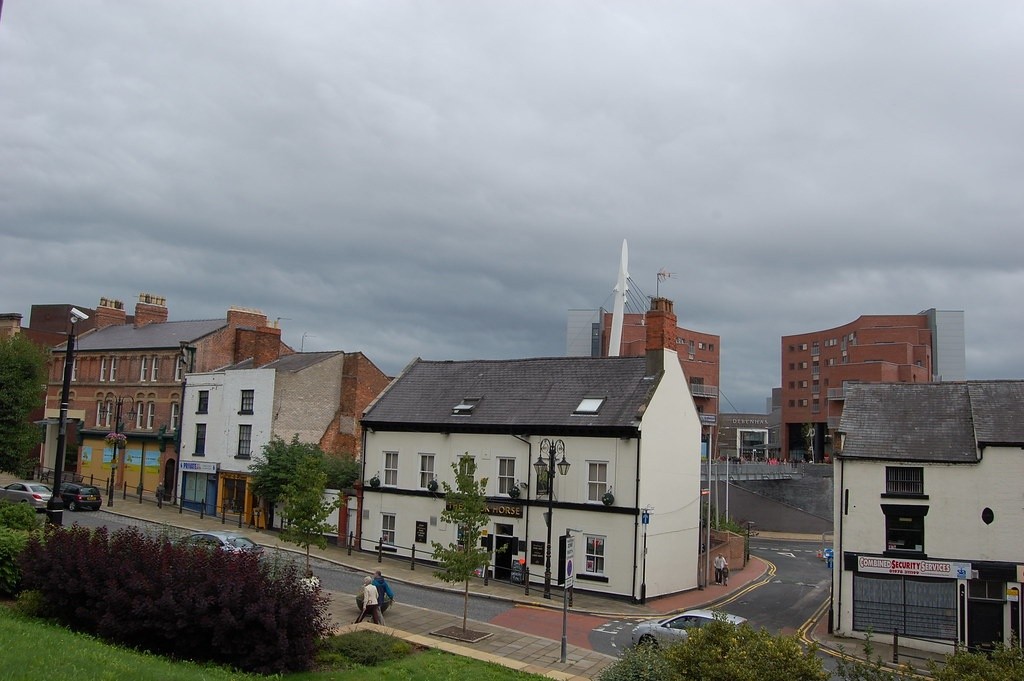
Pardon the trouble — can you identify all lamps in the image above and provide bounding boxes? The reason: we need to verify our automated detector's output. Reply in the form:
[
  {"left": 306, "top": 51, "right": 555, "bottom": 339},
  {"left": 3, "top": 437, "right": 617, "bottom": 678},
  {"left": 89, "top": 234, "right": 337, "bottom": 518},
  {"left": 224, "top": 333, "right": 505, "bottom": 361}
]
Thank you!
[
  {"left": 508, "top": 479, "right": 527, "bottom": 498},
  {"left": 602, "top": 485, "right": 614, "bottom": 505},
  {"left": 427, "top": 473, "right": 438, "bottom": 491},
  {"left": 370, "top": 470, "right": 380, "bottom": 488}
]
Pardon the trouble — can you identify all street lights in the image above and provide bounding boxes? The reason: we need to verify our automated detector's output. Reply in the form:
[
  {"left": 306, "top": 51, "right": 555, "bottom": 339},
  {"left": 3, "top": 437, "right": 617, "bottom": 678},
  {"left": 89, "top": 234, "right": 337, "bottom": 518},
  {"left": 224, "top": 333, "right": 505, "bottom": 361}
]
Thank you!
[
  {"left": 532, "top": 438, "right": 572, "bottom": 600},
  {"left": 747, "top": 521, "right": 755, "bottom": 561},
  {"left": 100, "top": 391, "right": 136, "bottom": 508}
]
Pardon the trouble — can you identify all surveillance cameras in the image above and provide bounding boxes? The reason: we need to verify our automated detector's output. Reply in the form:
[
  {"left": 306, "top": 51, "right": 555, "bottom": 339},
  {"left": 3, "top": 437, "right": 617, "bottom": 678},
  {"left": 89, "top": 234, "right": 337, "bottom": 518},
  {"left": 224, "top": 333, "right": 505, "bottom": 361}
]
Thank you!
[{"left": 69, "top": 307, "right": 90, "bottom": 321}]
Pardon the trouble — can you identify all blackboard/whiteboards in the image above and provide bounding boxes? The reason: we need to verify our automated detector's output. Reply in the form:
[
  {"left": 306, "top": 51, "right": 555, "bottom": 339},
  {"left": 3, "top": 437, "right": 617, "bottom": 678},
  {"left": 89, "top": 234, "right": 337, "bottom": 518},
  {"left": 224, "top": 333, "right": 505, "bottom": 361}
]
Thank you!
[{"left": 511, "top": 560, "right": 525, "bottom": 584}]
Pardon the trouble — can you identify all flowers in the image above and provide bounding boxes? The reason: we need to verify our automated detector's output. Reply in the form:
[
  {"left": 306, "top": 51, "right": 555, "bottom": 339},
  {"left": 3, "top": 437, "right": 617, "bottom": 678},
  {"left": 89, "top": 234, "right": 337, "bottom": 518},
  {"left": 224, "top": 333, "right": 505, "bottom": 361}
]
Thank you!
[{"left": 105, "top": 432, "right": 127, "bottom": 449}]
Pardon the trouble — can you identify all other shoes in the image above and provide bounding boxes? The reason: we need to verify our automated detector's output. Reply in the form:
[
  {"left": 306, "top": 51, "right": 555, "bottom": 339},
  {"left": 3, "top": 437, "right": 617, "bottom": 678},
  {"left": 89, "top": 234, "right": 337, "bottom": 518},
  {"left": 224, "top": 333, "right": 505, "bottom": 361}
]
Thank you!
[
  {"left": 715, "top": 582, "right": 721, "bottom": 585},
  {"left": 724, "top": 583, "right": 727, "bottom": 587}
]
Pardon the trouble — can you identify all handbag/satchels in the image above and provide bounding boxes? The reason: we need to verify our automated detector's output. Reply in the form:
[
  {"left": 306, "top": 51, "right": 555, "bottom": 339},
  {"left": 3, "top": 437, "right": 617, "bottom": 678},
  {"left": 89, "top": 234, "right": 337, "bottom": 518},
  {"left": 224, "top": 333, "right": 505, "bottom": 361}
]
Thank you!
[{"left": 156, "top": 492, "right": 161, "bottom": 497}]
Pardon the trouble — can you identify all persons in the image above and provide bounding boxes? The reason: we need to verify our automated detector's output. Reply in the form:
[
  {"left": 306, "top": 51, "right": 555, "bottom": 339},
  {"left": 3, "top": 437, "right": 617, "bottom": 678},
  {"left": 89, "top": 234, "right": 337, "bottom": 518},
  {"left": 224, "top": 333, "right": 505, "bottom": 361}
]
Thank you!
[
  {"left": 721, "top": 563, "right": 730, "bottom": 586},
  {"left": 714, "top": 553, "right": 726, "bottom": 585},
  {"left": 371, "top": 571, "right": 395, "bottom": 626},
  {"left": 155, "top": 482, "right": 165, "bottom": 509},
  {"left": 717, "top": 456, "right": 813, "bottom": 465},
  {"left": 355, "top": 576, "right": 379, "bottom": 624}
]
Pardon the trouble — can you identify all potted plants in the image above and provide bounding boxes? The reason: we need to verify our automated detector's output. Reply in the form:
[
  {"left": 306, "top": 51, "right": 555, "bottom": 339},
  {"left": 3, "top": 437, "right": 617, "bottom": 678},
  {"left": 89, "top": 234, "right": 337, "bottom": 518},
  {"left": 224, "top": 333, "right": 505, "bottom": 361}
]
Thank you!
[{"left": 356, "top": 585, "right": 390, "bottom": 616}]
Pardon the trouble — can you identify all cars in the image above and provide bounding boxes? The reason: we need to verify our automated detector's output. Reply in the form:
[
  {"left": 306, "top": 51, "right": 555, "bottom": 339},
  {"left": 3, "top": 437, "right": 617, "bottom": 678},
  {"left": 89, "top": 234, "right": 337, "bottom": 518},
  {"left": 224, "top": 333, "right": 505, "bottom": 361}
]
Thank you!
[
  {"left": 0, "top": 481, "right": 54, "bottom": 512},
  {"left": 167, "top": 530, "right": 265, "bottom": 563},
  {"left": 631, "top": 609, "right": 748, "bottom": 653}
]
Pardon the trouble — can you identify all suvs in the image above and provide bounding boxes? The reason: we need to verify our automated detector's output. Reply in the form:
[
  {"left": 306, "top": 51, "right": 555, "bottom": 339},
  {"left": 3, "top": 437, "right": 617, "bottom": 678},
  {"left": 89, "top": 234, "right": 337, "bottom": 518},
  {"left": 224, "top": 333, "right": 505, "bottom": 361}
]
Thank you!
[{"left": 54, "top": 482, "right": 102, "bottom": 512}]
